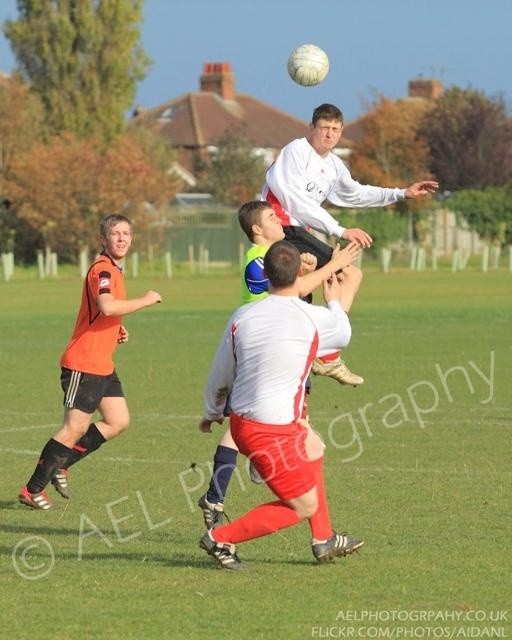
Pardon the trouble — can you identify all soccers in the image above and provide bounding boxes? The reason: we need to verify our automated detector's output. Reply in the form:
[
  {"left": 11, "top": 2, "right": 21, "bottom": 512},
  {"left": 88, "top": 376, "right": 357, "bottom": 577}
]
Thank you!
[{"left": 287, "top": 44, "right": 329, "bottom": 85}]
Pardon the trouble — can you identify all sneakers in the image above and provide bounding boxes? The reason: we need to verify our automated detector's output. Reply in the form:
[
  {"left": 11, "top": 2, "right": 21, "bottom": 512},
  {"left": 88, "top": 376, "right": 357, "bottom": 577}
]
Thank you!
[
  {"left": 195, "top": 491, "right": 225, "bottom": 529},
  {"left": 50, "top": 464, "right": 72, "bottom": 499},
  {"left": 197, "top": 524, "right": 244, "bottom": 573},
  {"left": 310, "top": 528, "right": 365, "bottom": 564},
  {"left": 17, "top": 485, "right": 57, "bottom": 512},
  {"left": 311, "top": 353, "right": 366, "bottom": 389}
]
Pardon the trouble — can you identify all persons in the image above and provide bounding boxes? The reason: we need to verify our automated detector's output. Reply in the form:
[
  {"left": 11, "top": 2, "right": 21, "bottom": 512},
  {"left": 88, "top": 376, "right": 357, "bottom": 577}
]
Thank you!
[
  {"left": 18, "top": 213, "right": 162, "bottom": 510},
  {"left": 260, "top": 104, "right": 439, "bottom": 389},
  {"left": 198, "top": 200, "right": 363, "bottom": 531},
  {"left": 199, "top": 239, "right": 366, "bottom": 570}
]
